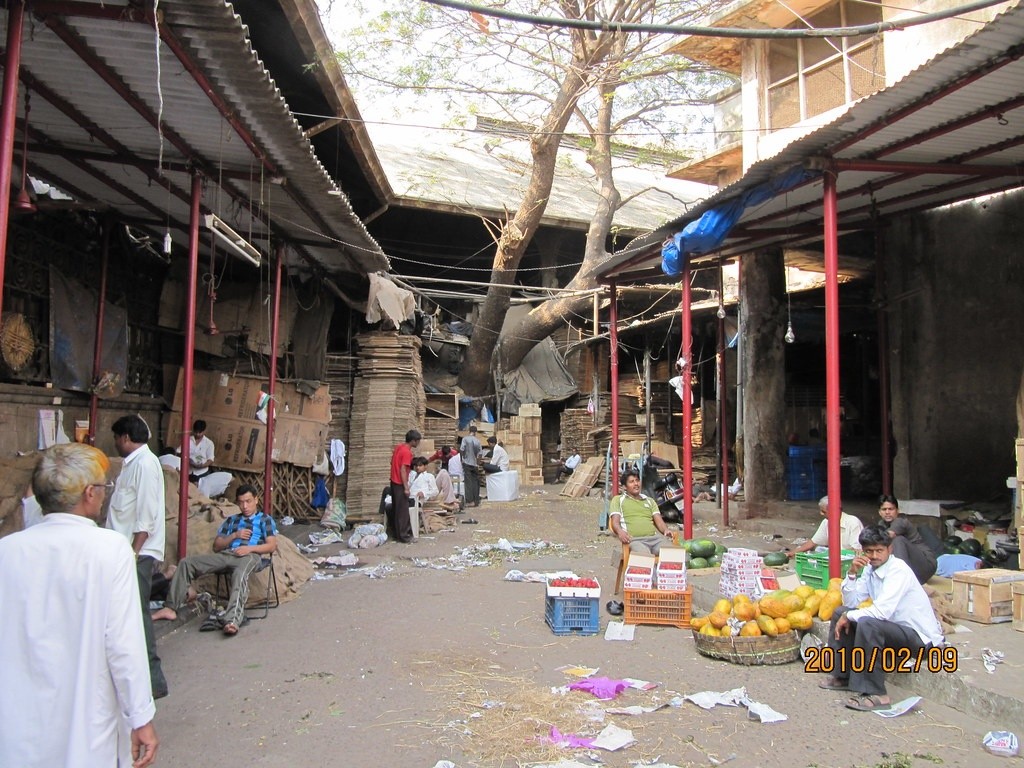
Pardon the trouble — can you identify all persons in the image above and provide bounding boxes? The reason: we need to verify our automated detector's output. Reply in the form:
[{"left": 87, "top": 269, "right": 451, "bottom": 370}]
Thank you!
[
  {"left": 158, "top": 419, "right": 216, "bottom": 483},
  {"left": 389, "top": 429, "right": 439, "bottom": 543},
  {"left": 552, "top": 447, "right": 581, "bottom": 485},
  {"left": 0, "top": 442, "right": 159, "bottom": 768},
  {"left": 609, "top": 469, "right": 674, "bottom": 557},
  {"left": 151, "top": 484, "right": 277, "bottom": 636},
  {"left": 428, "top": 426, "right": 483, "bottom": 507},
  {"left": 20, "top": 412, "right": 197, "bottom": 701},
  {"left": 876, "top": 494, "right": 938, "bottom": 585},
  {"left": 482, "top": 435, "right": 510, "bottom": 473},
  {"left": 817, "top": 524, "right": 945, "bottom": 712},
  {"left": 692, "top": 477, "right": 742, "bottom": 503},
  {"left": 784, "top": 495, "right": 865, "bottom": 557}
]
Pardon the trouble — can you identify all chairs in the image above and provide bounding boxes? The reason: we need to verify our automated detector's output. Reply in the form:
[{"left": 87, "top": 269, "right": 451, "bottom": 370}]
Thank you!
[
  {"left": 213, "top": 548, "right": 280, "bottom": 620},
  {"left": 383, "top": 495, "right": 429, "bottom": 538}
]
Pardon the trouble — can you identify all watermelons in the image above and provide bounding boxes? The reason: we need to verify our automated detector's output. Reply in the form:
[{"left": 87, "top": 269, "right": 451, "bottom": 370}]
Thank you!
[
  {"left": 763, "top": 552, "right": 790, "bottom": 566},
  {"left": 942, "top": 535, "right": 990, "bottom": 561},
  {"left": 677, "top": 537, "right": 729, "bottom": 569}
]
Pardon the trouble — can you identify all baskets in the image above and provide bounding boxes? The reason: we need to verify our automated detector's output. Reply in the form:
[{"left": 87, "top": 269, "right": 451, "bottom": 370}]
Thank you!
[{"left": 690, "top": 628, "right": 802, "bottom": 665}]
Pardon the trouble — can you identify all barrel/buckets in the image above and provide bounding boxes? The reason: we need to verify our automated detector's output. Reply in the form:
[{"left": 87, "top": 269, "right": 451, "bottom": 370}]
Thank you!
[{"left": 975, "top": 523, "right": 1001, "bottom": 534}]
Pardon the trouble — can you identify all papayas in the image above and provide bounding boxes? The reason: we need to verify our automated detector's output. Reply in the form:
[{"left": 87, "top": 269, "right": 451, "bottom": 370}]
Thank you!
[{"left": 690, "top": 578, "right": 873, "bottom": 637}]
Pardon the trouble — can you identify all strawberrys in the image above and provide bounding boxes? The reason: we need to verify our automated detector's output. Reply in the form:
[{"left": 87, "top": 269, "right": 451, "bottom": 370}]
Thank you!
[{"left": 550, "top": 577, "right": 598, "bottom": 587}]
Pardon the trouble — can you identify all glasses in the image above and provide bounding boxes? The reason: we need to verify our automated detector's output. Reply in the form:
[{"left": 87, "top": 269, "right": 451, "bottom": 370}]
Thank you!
[{"left": 84, "top": 479, "right": 115, "bottom": 495}]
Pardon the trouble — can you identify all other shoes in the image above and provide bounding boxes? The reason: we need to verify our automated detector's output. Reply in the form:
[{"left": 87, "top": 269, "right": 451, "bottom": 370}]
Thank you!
[
  {"left": 558, "top": 479, "right": 565, "bottom": 483},
  {"left": 200, "top": 613, "right": 249, "bottom": 630},
  {"left": 465, "top": 501, "right": 475, "bottom": 507},
  {"left": 551, "top": 481, "right": 559, "bottom": 485}
]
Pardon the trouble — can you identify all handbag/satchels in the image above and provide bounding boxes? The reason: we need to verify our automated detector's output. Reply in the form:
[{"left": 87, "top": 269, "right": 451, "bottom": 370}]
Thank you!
[{"left": 480, "top": 404, "right": 494, "bottom": 423}]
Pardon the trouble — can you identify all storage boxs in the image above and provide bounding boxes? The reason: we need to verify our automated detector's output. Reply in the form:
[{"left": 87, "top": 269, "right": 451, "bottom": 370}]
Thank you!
[
  {"left": 952, "top": 438, "right": 1024, "bottom": 623},
  {"left": 785, "top": 445, "right": 850, "bottom": 500},
  {"left": 718, "top": 548, "right": 763, "bottom": 602},
  {"left": 795, "top": 550, "right": 864, "bottom": 589},
  {"left": 544, "top": 575, "right": 601, "bottom": 637},
  {"left": 624, "top": 547, "right": 691, "bottom": 629}
]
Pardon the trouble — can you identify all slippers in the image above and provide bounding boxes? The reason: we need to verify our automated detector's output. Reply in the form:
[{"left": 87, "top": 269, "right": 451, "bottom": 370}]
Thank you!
[
  {"left": 462, "top": 518, "right": 479, "bottom": 524},
  {"left": 846, "top": 693, "right": 892, "bottom": 711},
  {"left": 818, "top": 675, "right": 851, "bottom": 690}
]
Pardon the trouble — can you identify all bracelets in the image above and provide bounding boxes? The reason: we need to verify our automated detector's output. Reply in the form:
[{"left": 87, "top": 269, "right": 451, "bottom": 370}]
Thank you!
[{"left": 848, "top": 571, "right": 857, "bottom": 580}]
